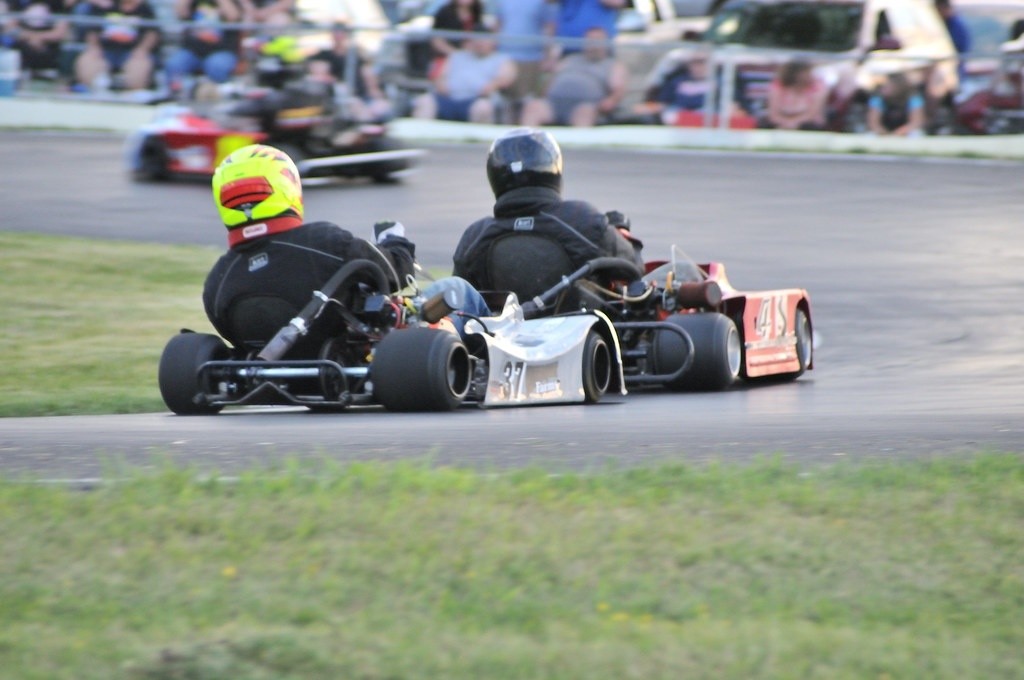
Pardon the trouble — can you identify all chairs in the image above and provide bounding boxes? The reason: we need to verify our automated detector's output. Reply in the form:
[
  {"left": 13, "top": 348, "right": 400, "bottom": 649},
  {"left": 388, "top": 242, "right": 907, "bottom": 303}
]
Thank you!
[
  {"left": 489, "top": 230, "right": 572, "bottom": 305},
  {"left": 230, "top": 297, "right": 323, "bottom": 357}
]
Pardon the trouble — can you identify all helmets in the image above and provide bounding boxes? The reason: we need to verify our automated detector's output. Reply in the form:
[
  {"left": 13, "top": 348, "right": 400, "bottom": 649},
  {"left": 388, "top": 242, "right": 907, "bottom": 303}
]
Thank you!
[
  {"left": 486, "top": 127, "right": 564, "bottom": 201},
  {"left": 212, "top": 144, "right": 304, "bottom": 248}
]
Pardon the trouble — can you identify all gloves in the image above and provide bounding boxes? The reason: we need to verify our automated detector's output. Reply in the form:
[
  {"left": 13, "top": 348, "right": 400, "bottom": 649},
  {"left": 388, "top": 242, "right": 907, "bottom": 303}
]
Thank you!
[
  {"left": 607, "top": 210, "right": 629, "bottom": 231},
  {"left": 373, "top": 220, "right": 405, "bottom": 243}
]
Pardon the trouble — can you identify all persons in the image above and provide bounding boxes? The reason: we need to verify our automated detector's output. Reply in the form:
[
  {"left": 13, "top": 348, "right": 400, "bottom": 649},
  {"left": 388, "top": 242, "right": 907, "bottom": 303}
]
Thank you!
[
  {"left": 0, "top": 0, "right": 1024, "bottom": 155},
  {"left": 202, "top": 144, "right": 493, "bottom": 347},
  {"left": 452, "top": 128, "right": 709, "bottom": 311}
]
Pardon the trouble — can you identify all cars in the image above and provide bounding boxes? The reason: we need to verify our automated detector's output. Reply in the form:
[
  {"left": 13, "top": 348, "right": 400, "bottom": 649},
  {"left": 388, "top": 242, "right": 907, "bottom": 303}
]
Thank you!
[
  {"left": 643, "top": 0, "right": 959, "bottom": 121},
  {"left": 368, "top": 0, "right": 675, "bottom": 92}
]
